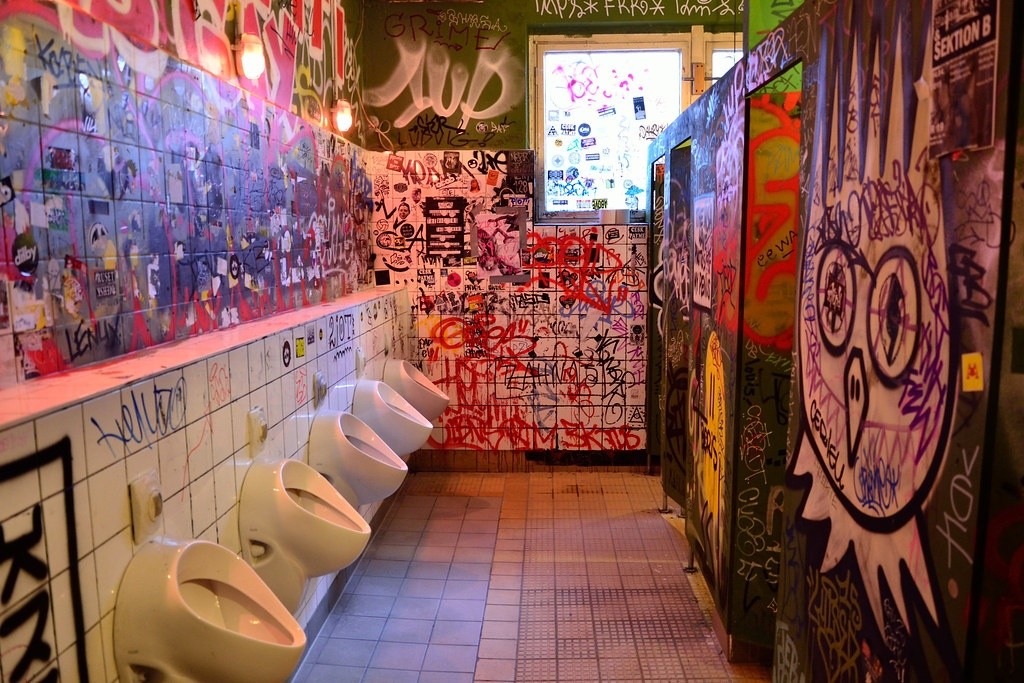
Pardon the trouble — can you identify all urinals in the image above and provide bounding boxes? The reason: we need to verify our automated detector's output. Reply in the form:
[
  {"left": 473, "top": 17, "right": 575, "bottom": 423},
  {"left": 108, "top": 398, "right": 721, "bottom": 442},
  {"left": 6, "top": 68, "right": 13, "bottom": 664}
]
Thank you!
[
  {"left": 309, "top": 411, "right": 408, "bottom": 506},
  {"left": 352, "top": 381, "right": 433, "bottom": 457},
  {"left": 384, "top": 358, "right": 450, "bottom": 421},
  {"left": 114, "top": 537, "right": 307, "bottom": 683},
  {"left": 239, "top": 459, "right": 371, "bottom": 579}
]
